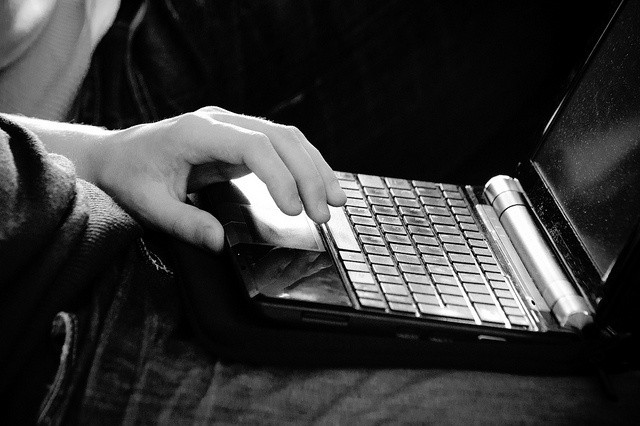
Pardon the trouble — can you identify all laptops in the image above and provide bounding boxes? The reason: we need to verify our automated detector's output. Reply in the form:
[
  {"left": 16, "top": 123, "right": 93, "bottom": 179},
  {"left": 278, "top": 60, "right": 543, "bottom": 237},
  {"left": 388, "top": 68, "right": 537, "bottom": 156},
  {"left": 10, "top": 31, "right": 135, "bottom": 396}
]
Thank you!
[
  {"left": 634, "top": 371, "right": 636, "bottom": 371},
  {"left": 197, "top": 2, "right": 635, "bottom": 373}
]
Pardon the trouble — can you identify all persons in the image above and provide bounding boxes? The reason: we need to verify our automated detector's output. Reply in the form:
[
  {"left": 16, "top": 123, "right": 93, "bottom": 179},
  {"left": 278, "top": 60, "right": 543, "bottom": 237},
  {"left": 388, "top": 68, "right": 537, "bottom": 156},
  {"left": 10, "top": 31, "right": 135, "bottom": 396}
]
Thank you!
[{"left": 0, "top": 103, "right": 631, "bottom": 425}]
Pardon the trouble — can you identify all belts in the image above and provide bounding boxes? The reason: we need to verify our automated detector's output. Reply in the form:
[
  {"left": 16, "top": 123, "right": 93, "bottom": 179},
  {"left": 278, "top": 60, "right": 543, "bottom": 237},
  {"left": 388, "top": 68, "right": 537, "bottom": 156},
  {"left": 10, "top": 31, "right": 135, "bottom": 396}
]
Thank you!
[{"left": 81, "top": 258, "right": 146, "bottom": 426}]
